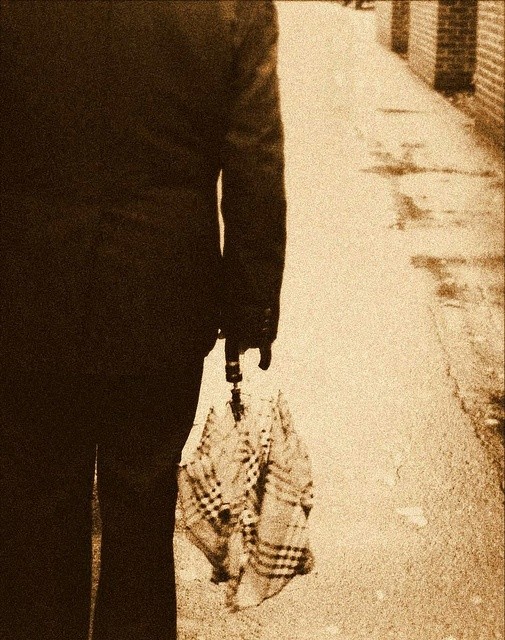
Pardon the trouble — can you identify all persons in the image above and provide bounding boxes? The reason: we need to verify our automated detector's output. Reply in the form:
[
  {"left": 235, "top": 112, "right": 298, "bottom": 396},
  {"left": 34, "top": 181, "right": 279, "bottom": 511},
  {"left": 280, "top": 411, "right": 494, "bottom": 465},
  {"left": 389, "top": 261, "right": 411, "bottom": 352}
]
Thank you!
[{"left": 1, "top": 1, "right": 287, "bottom": 639}]
